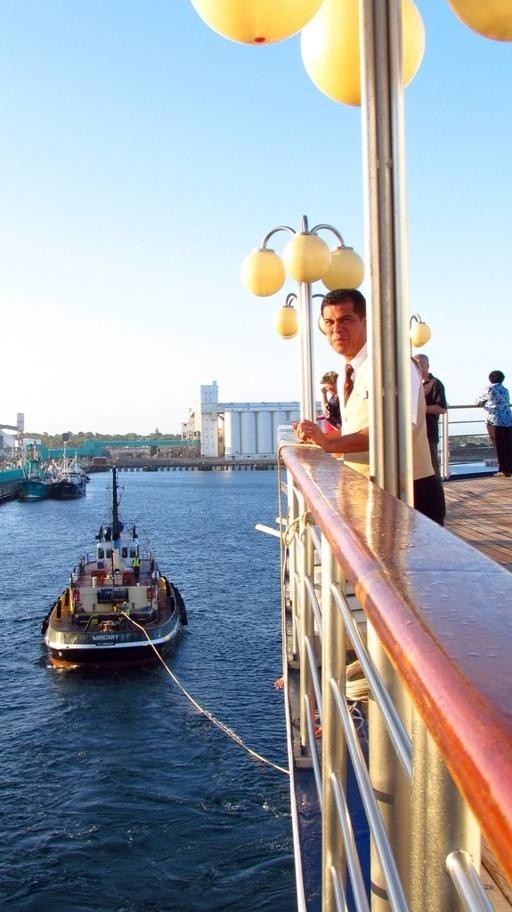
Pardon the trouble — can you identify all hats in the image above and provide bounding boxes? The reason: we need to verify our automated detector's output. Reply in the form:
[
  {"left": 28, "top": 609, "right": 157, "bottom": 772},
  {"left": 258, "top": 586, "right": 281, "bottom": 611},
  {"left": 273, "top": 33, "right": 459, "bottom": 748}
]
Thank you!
[{"left": 320, "top": 370, "right": 338, "bottom": 384}]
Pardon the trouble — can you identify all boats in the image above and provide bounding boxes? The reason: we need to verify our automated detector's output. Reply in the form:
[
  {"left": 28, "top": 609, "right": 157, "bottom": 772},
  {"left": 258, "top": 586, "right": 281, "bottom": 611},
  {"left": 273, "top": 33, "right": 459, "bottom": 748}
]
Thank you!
[{"left": 18, "top": 442, "right": 90, "bottom": 501}]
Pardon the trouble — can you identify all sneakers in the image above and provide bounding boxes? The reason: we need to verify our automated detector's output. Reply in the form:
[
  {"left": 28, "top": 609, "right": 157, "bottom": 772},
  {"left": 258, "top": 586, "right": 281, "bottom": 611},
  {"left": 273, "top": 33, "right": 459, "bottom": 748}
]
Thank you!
[{"left": 494, "top": 471, "right": 511, "bottom": 477}]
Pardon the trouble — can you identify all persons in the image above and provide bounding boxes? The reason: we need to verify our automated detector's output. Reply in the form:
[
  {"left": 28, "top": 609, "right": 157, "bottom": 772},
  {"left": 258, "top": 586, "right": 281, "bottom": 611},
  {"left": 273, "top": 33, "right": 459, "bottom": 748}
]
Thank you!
[
  {"left": 117, "top": 601, "right": 132, "bottom": 629},
  {"left": 273, "top": 677, "right": 284, "bottom": 690},
  {"left": 296, "top": 288, "right": 446, "bottom": 527},
  {"left": 414, "top": 354, "right": 447, "bottom": 486},
  {"left": 474, "top": 371, "right": 512, "bottom": 477},
  {"left": 320, "top": 371, "right": 342, "bottom": 457},
  {"left": 132, "top": 557, "right": 140, "bottom": 586}
]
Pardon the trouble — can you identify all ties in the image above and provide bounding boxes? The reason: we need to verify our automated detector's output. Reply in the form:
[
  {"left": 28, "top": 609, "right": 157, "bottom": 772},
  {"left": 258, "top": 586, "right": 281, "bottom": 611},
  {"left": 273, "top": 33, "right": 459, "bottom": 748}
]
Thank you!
[{"left": 344, "top": 364, "right": 355, "bottom": 406}]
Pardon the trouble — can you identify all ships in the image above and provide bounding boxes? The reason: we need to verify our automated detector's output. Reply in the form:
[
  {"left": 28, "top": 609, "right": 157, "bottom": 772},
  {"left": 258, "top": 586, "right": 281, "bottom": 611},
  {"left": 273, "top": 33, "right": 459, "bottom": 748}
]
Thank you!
[{"left": 42, "top": 465, "right": 188, "bottom": 673}]
